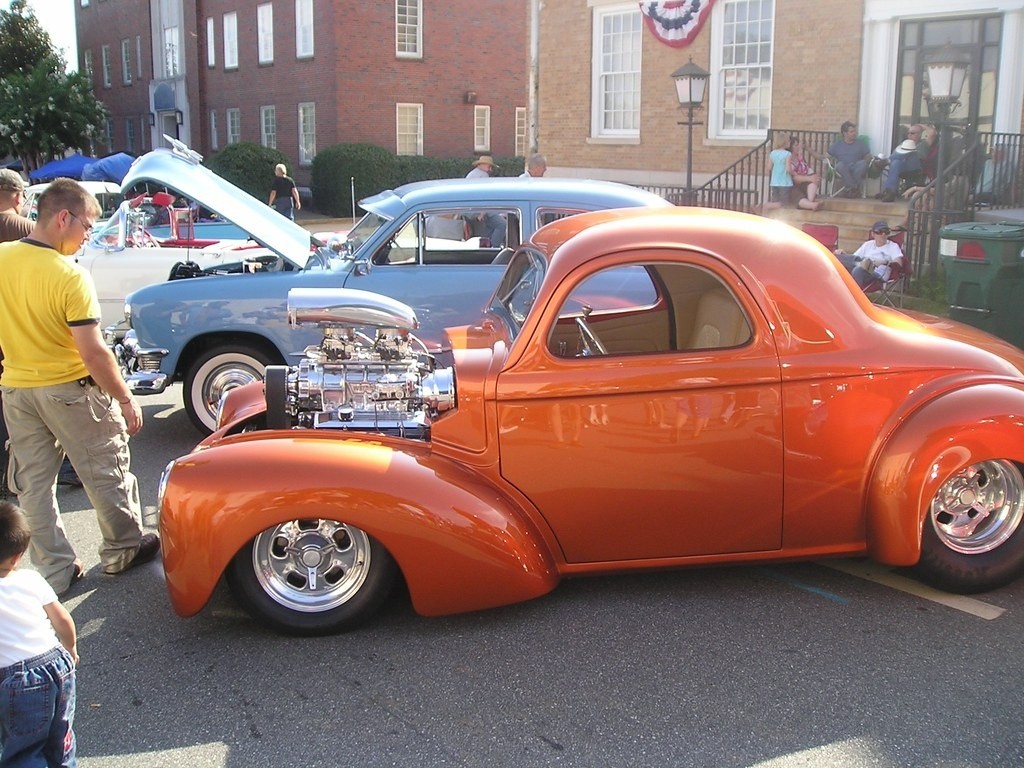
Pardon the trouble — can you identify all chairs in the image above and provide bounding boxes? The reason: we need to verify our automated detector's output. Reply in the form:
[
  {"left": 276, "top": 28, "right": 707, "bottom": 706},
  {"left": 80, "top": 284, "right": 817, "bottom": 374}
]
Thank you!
[
  {"left": 822, "top": 134, "right": 874, "bottom": 199},
  {"left": 685, "top": 286, "right": 753, "bottom": 348},
  {"left": 801, "top": 222, "right": 846, "bottom": 255},
  {"left": 858, "top": 230, "right": 911, "bottom": 310}
]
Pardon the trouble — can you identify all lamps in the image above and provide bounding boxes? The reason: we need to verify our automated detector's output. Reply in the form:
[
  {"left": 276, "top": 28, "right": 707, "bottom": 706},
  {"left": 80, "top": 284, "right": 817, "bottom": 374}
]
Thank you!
[
  {"left": 175, "top": 111, "right": 183, "bottom": 125},
  {"left": 147, "top": 112, "right": 155, "bottom": 126}
]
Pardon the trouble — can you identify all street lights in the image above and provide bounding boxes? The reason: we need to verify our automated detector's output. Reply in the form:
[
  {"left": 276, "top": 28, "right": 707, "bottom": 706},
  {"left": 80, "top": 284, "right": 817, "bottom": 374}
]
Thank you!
[
  {"left": 670, "top": 55, "right": 712, "bottom": 206},
  {"left": 920, "top": 36, "right": 971, "bottom": 289}
]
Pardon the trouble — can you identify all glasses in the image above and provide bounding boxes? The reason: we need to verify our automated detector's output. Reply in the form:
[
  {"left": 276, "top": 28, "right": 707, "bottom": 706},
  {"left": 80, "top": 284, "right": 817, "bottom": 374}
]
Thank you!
[
  {"left": 906, "top": 131, "right": 919, "bottom": 135},
  {"left": 873, "top": 229, "right": 890, "bottom": 236},
  {"left": 68, "top": 210, "right": 94, "bottom": 237}
]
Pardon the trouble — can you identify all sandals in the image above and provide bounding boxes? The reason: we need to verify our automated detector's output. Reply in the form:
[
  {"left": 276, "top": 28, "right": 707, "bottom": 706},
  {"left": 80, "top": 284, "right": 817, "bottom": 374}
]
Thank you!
[
  {"left": 891, "top": 225, "right": 908, "bottom": 231},
  {"left": 912, "top": 222, "right": 930, "bottom": 236}
]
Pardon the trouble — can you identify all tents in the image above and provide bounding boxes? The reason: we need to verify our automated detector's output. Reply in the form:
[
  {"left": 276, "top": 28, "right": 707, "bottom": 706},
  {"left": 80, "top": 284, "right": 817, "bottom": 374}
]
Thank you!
[{"left": 0, "top": 152, "right": 137, "bottom": 219}]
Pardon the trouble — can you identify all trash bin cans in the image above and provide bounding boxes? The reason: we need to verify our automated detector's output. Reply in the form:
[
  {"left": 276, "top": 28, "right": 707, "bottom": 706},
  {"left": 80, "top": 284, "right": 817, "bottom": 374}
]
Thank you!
[{"left": 939, "top": 221, "right": 1024, "bottom": 349}]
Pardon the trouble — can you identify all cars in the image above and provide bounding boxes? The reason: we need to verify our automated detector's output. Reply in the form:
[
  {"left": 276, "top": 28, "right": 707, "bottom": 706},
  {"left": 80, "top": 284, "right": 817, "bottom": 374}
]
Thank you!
[
  {"left": 19, "top": 182, "right": 123, "bottom": 241},
  {"left": 155, "top": 204, "right": 1024, "bottom": 638},
  {"left": 102, "top": 129, "right": 686, "bottom": 437},
  {"left": 72, "top": 199, "right": 281, "bottom": 342}
]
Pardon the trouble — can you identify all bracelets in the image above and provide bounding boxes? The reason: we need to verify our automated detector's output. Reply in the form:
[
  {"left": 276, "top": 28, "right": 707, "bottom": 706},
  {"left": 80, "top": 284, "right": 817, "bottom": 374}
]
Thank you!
[{"left": 119, "top": 394, "right": 134, "bottom": 404}]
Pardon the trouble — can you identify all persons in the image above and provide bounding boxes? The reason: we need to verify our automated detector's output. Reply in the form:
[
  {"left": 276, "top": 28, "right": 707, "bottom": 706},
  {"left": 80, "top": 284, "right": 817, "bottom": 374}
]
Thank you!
[
  {"left": 765, "top": 121, "right": 1010, "bottom": 235},
  {"left": 267, "top": 164, "right": 301, "bottom": 223},
  {"left": 3, "top": 180, "right": 160, "bottom": 597},
  {"left": 0, "top": 504, "right": 79, "bottom": 768},
  {"left": 834, "top": 222, "right": 904, "bottom": 291},
  {"left": 466, "top": 156, "right": 507, "bottom": 248},
  {"left": 0, "top": 169, "right": 85, "bottom": 499},
  {"left": 519, "top": 154, "right": 548, "bottom": 177}
]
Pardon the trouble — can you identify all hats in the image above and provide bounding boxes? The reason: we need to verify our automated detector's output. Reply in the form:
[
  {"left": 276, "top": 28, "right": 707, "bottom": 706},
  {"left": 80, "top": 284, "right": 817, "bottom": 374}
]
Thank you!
[
  {"left": 989, "top": 141, "right": 1008, "bottom": 153},
  {"left": 472, "top": 156, "right": 499, "bottom": 168},
  {"left": 862, "top": 153, "right": 882, "bottom": 179},
  {"left": 0, "top": 168, "right": 27, "bottom": 199},
  {"left": 896, "top": 139, "right": 918, "bottom": 154},
  {"left": 872, "top": 221, "right": 891, "bottom": 231}
]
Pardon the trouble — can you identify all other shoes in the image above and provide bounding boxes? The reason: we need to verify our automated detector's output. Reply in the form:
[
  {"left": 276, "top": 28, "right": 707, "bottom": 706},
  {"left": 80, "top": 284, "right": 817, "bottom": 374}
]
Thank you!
[
  {"left": 753, "top": 203, "right": 763, "bottom": 216},
  {"left": 57, "top": 455, "right": 82, "bottom": 487},
  {"left": 105, "top": 532, "right": 161, "bottom": 574},
  {"left": 862, "top": 258, "right": 875, "bottom": 273},
  {"left": 847, "top": 186, "right": 862, "bottom": 198},
  {"left": 57, "top": 559, "right": 84, "bottom": 599},
  {"left": 882, "top": 191, "right": 896, "bottom": 202}
]
